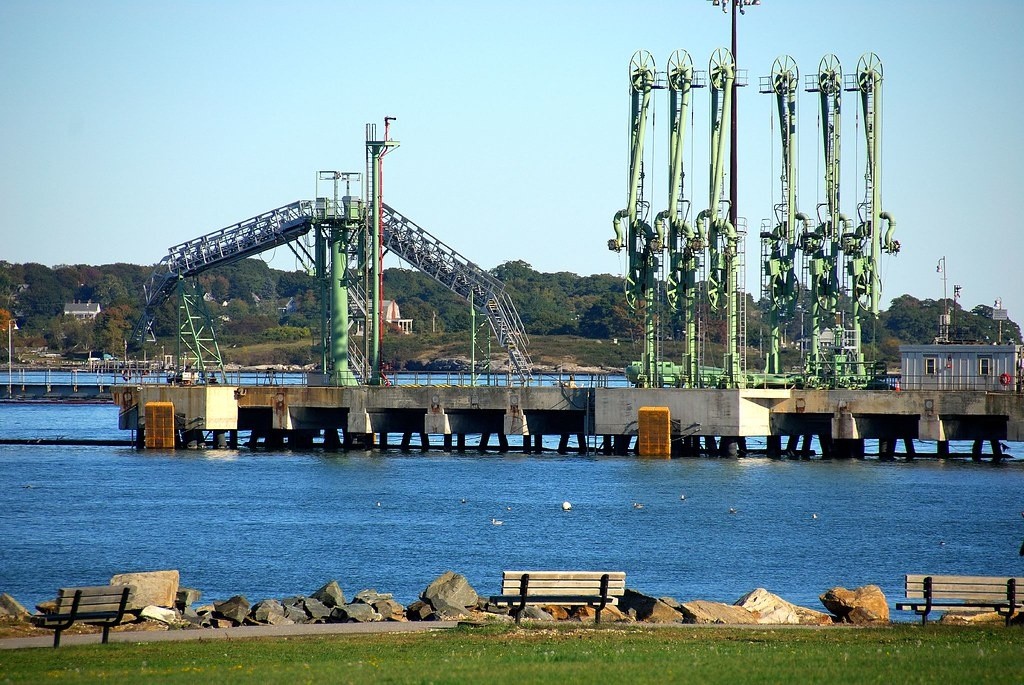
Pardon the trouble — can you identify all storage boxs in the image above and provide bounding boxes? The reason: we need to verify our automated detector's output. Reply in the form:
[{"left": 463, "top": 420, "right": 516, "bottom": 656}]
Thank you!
[
  {"left": 144, "top": 402, "right": 175, "bottom": 449},
  {"left": 637, "top": 407, "right": 671, "bottom": 456}
]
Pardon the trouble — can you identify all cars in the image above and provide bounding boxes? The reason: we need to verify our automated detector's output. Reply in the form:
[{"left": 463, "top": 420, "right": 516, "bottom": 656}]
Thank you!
[{"left": 862, "top": 374, "right": 901, "bottom": 390}]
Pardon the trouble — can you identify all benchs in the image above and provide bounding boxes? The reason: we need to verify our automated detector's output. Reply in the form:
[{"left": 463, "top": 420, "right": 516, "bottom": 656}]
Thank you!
[
  {"left": 535, "top": 371, "right": 615, "bottom": 388},
  {"left": 28, "top": 584, "right": 137, "bottom": 648},
  {"left": 897, "top": 572, "right": 1024, "bottom": 631},
  {"left": 489, "top": 570, "right": 626, "bottom": 628}
]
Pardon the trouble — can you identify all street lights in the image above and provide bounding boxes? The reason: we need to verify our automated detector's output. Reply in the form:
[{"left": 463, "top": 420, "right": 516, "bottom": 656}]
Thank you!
[
  {"left": 937, "top": 255, "right": 948, "bottom": 343},
  {"left": 8, "top": 319, "right": 20, "bottom": 398},
  {"left": 994, "top": 296, "right": 1003, "bottom": 345}
]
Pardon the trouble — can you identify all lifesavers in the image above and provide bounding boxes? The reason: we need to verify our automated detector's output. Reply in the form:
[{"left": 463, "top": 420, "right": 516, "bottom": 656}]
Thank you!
[{"left": 1000, "top": 372, "right": 1011, "bottom": 386}]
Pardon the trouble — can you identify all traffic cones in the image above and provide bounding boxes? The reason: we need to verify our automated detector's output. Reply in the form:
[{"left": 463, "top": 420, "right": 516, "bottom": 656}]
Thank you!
[{"left": 895, "top": 380, "right": 901, "bottom": 391}]
